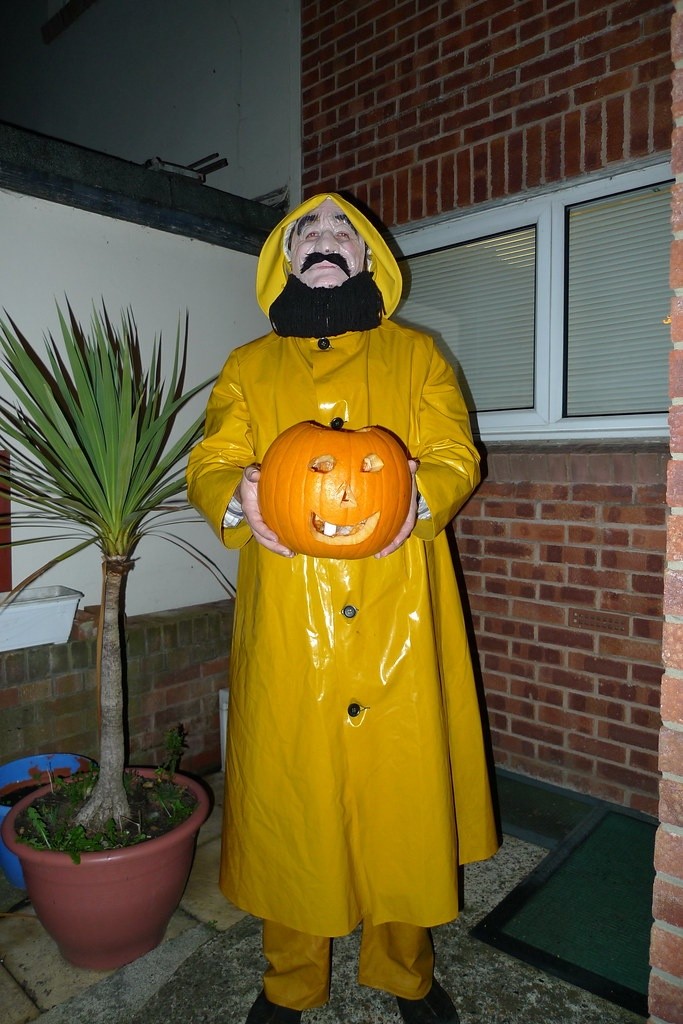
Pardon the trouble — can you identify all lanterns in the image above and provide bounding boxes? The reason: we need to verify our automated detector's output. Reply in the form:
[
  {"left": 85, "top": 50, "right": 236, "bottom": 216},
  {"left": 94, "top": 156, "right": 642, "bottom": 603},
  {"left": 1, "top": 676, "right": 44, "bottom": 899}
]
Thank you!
[{"left": 257, "top": 420, "right": 412, "bottom": 561}]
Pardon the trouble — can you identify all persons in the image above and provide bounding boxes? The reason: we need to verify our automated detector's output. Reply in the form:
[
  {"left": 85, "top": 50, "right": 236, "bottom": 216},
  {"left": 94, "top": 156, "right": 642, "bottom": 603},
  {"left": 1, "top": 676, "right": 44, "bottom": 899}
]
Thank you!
[{"left": 184, "top": 189, "right": 502, "bottom": 1024}]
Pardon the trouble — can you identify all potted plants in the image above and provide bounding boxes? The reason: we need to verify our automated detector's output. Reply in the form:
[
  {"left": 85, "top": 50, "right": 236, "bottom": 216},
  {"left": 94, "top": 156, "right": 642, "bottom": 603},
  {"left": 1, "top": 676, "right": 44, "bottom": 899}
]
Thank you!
[{"left": 0, "top": 291, "right": 236, "bottom": 975}]
